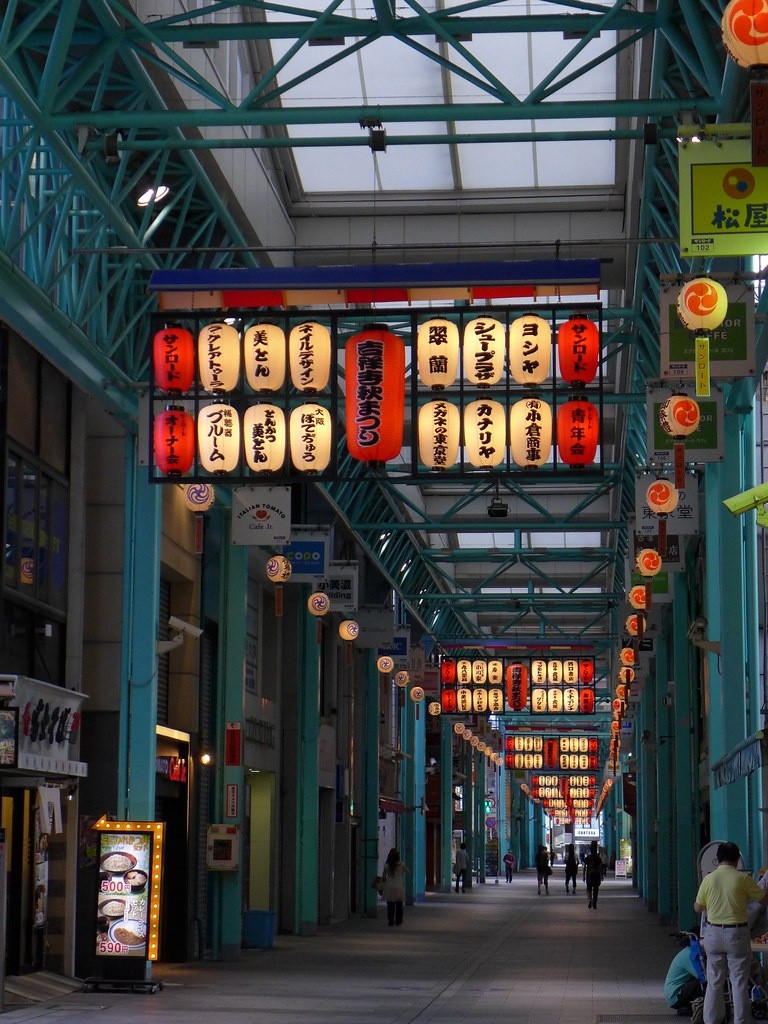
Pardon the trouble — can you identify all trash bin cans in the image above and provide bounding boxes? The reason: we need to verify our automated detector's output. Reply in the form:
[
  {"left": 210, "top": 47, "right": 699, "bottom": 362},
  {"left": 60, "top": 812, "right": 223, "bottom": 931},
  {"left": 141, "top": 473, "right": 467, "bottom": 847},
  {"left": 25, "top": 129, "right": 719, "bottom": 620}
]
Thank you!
[{"left": 242, "top": 910, "right": 278, "bottom": 950}]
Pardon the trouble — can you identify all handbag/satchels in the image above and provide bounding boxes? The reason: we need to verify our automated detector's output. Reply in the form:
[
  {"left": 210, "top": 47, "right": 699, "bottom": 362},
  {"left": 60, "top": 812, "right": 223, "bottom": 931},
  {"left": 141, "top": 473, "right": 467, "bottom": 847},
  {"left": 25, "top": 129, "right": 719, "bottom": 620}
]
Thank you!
[{"left": 453, "top": 863, "right": 461, "bottom": 874}]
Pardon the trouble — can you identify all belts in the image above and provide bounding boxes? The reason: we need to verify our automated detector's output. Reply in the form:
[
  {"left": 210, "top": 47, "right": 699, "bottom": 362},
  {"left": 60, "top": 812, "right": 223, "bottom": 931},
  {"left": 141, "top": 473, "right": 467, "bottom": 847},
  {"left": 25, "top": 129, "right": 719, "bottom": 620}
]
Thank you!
[{"left": 708, "top": 922, "right": 747, "bottom": 928}]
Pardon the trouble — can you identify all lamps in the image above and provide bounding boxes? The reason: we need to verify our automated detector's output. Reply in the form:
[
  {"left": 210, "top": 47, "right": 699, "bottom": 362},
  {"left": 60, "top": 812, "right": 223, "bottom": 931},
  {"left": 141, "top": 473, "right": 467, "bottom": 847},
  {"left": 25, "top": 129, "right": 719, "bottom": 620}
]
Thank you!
[
  {"left": 488, "top": 502, "right": 510, "bottom": 518},
  {"left": 413, "top": 795, "right": 430, "bottom": 815},
  {"left": 367, "top": 128, "right": 387, "bottom": 154},
  {"left": 67, "top": 787, "right": 78, "bottom": 801},
  {"left": 506, "top": 599, "right": 521, "bottom": 609},
  {"left": 131, "top": 155, "right": 170, "bottom": 206},
  {"left": 675, "top": 103, "right": 704, "bottom": 142}
]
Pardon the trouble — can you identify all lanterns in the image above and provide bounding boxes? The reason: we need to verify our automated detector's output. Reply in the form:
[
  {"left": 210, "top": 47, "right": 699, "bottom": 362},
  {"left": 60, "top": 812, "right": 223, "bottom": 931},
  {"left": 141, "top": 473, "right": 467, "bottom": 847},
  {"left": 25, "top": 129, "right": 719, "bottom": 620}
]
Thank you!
[
  {"left": 442, "top": 659, "right": 594, "bottom": 713},
  {"left": 505, "top": 736, "right": 598, "bottom": 770},
  {"left": 721, "top": 0, "right": 768, "bottom": 70},
  {"left": 153, "top": 313, "right": 600, "bottom": 476},
  {"left": 532, "top": 775, "right": 595, "bottom": 824},
  {"left": 596, "top": 393, "right": 700, "bottom": 813},
  {"left": 185, "top": 484, "right": 540, "bottom": 803},
  {"left": 677, "top": 274, "right": 728, "bottom": 333}
]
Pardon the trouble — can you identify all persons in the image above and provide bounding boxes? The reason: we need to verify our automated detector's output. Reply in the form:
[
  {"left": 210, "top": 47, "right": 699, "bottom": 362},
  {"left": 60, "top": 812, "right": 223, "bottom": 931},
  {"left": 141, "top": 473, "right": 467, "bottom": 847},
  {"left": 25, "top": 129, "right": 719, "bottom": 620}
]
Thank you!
[
  {"left": 582, "top": 845, "right": 603, "bottom": 909},
  {"left": 564, "top": 844, "right": 579, "bottom": 896},
  {"left": 455, "top": 843, "right": 468, "bottom": 893},
  {"left": 503, "top": 851, "right": 514, "bottom": 883},
  {"left": 535, "top": 846, "right": 551, "bottom": 896},
  {"left": 601, "top": 852, "right": 607, "bottom": 881},
  {"left": 580, "top": 850, "right": 585, "bottom": 863},
  {"left": 540, "top": 847, "right": 551, "bottom": 884},
  {"left": 663, "top": 945, "right": 707, "bottom": 1017},
  {"left": 550, "top": 849, "right": 555, "bottom": 867},
  {"left": 693, "top": 841, "right": 768, "bottom": 1024},
  {"left": 382, "top": 847, "right": 410, "bottom": 926}
]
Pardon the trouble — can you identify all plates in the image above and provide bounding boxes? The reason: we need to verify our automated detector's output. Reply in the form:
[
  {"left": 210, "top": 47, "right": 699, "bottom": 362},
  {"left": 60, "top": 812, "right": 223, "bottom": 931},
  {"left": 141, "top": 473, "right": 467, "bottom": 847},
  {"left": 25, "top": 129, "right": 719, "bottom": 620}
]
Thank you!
[{"left": 108, "top": 918, "right": 148, "bottom": 951}]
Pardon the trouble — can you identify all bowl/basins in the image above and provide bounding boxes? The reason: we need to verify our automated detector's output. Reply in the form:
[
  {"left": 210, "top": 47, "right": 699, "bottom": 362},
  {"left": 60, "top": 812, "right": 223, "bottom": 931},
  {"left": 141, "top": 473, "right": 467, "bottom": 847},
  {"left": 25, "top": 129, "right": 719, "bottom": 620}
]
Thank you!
[
  {"left": 122, "top": 870, "right": 148, "bottom": 893},
  {"left": 99, "top": 851, "right": 138, "bottom": 876},
  {"left": 98, "top": 899, "right": 131, "bottom": 920}
]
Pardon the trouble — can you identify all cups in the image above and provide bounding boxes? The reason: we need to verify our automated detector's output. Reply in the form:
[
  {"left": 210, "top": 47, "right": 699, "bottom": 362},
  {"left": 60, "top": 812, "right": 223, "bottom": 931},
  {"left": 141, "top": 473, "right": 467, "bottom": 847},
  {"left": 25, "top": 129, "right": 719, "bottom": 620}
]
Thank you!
[{"left": 96, "top": 916, "right": 110, "bottom": 934}]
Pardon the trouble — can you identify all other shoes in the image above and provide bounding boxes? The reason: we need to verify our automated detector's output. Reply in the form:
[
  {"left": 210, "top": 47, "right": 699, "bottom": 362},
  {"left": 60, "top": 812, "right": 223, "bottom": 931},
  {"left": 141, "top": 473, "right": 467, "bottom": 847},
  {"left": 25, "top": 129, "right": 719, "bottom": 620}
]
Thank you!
[
  {"left": 389, "top": 921, "right": 394, "bottom": 926},
  {"left": 588, "top": 901, "right": 591, "bottom": 908},
  {"left": 397, "top": 920, "right": 402, "bottom": 925},
  {"left": 677, "top": 1007, "right": 693, "bottom": 1016},
  {"left": 594, "top": 906, "right": 597, "bottom": 909}
]
